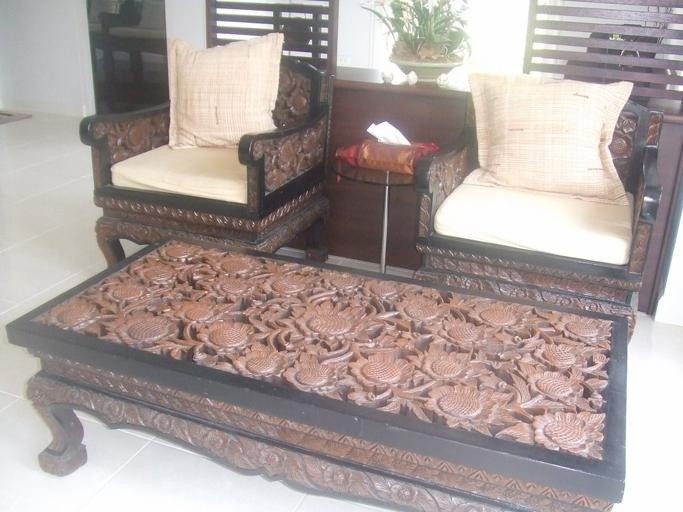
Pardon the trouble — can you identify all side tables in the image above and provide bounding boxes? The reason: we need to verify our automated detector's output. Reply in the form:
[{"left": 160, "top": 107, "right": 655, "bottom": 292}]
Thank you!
[{"left": 332, "top": 160, "right": 421, "bottom": 275}]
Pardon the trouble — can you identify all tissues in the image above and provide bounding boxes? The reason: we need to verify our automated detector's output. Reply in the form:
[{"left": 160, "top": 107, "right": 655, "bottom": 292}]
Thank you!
[{"left": 333, "top": 119, "right": 440, "bottom": 177}]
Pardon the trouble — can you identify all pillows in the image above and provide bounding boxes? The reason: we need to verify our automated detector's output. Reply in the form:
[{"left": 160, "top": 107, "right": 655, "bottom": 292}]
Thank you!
[
  {"left": 463, "top": 72, "right": 634, "bottom": 206},
  {"left": 167, "top": 32, "right": 284, "bottom": 149}
]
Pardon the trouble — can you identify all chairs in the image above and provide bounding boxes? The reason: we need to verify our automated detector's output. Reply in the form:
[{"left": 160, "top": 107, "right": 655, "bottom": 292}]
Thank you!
[
  {"left": 414, "top": 101, "right": 683, "bottom": 318},
  {"left": 79, "top": 56, "right": 329, "bottom": 267}
]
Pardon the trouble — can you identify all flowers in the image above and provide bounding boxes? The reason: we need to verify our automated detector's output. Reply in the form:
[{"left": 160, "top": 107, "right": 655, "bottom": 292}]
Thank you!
[{"left": 360, "top": 0, "right": 469, "bottom": 59}]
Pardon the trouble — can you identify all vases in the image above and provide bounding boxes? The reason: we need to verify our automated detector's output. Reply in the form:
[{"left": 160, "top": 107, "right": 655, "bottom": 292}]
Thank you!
[{"left": 390, "top": 56, "right": 462, "bottom": 84}]
